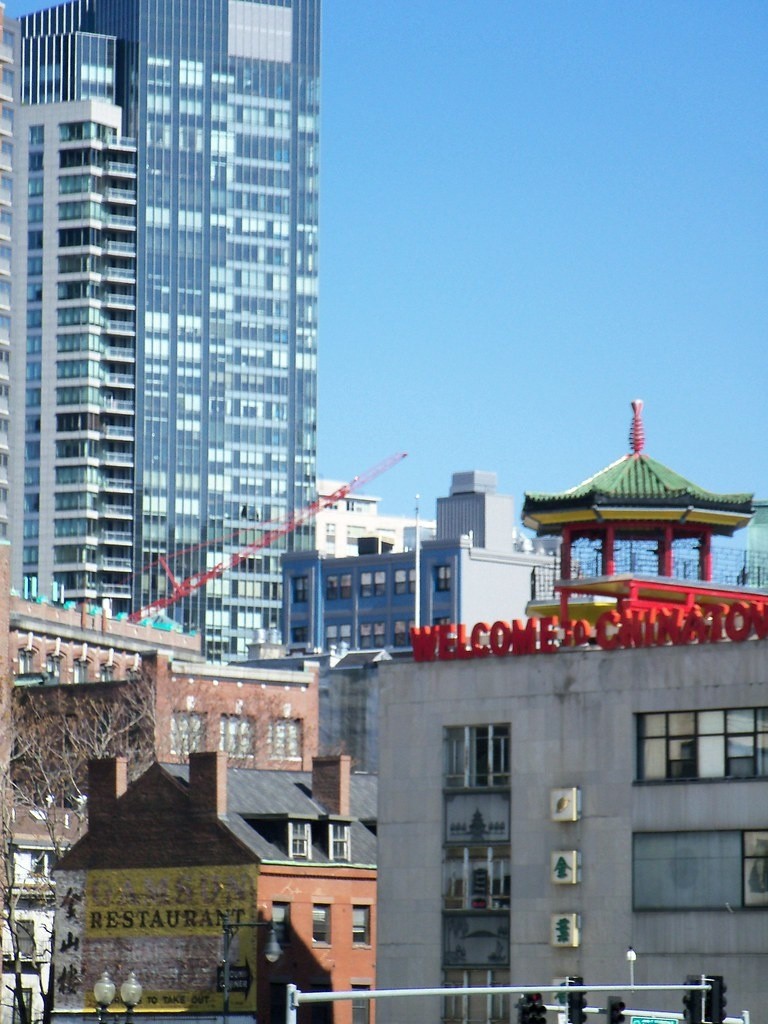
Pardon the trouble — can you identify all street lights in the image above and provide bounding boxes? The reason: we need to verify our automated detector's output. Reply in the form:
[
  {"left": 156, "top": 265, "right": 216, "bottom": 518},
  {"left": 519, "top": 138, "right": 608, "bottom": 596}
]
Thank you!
[{"left": 221, "top": 918, "right": 281, "bottom": 1024}]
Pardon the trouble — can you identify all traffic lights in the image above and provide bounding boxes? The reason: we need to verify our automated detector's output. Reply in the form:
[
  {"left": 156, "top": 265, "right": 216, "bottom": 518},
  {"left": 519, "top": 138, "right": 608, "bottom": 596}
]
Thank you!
[
  {"left": 608, "top": 996, "right": 625, "bottom": 1024},
  {"left": 569, "top": 977, "right": 587, "bottom": 1024},
  {"left": 521, "top": 993, "right": 547, "bottom": 1024}
]
